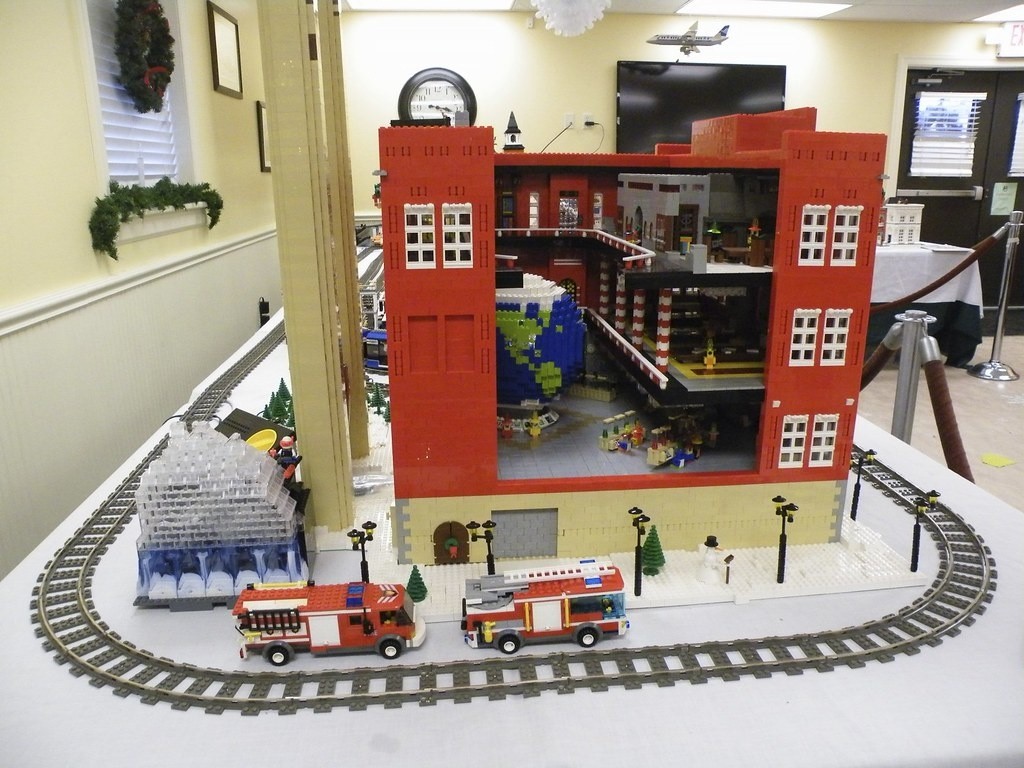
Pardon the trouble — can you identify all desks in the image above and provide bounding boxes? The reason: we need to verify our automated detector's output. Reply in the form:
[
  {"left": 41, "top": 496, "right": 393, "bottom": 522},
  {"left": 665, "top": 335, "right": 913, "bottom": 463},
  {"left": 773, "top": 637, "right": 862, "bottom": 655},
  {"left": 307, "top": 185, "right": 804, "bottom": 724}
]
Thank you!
[{"left": 865, "top": 244, "right": 984, "bottom": 369}]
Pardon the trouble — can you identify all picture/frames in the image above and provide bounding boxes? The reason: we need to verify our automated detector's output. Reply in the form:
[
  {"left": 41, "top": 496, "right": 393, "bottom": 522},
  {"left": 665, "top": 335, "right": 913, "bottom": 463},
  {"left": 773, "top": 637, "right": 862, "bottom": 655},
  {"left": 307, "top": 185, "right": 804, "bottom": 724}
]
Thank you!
[
  {"left": 206, "top": 0, "right": 244, "bottom": 99},
  {"left": 256, "top": 100, "right": 271, "bottom": 173}
]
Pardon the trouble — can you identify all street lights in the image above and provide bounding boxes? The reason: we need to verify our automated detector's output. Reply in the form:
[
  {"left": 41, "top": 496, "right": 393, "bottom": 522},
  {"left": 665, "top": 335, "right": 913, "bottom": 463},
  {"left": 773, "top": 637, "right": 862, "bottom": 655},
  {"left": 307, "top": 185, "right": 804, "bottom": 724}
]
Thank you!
[
  {"left": 772, "top": 493, "right": 799, "bottom": 584},
  {"left": 348, "top": 520, "right": 379, "bottom": 585},
  {"left": 850, "top": 448, "right": 878, "bottom": 521},
  {"left": 467, "top": 519, "right": 499, "bottom": 576},
  {"left": 911, "top": 489, "right": 941, "bottom": 574},
  {"left": 629, "top": 506, "right": 651, "bottom": 598}
]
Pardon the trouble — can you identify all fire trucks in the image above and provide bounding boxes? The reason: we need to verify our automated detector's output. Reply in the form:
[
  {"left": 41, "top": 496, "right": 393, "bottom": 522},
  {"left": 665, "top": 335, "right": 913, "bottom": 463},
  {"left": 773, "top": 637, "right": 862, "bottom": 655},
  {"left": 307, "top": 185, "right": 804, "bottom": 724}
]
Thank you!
[
  {"left": 460, "top": 559, "right": 628, "bottom": 654},
  {"left": 234, "top": 580, "right": 430, "bottom": 669}
]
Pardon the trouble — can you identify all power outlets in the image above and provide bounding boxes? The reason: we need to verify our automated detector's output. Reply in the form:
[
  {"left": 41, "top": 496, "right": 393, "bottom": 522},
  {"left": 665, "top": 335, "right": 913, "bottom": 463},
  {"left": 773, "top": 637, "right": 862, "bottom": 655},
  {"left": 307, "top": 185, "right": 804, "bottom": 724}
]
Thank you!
[
  {"left": 563, "top": 113, "right": 576, "bottom": 130},
  {"left": 583, "top": 113, "right": 595, "bottom": 129}
]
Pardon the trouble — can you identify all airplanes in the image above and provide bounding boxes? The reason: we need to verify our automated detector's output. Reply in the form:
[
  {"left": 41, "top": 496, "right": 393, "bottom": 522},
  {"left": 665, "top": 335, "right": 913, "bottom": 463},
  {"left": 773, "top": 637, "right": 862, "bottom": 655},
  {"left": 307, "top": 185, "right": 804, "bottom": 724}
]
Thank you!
[{"left": 645, "top": 20, "right": 731, "bottom": 57}]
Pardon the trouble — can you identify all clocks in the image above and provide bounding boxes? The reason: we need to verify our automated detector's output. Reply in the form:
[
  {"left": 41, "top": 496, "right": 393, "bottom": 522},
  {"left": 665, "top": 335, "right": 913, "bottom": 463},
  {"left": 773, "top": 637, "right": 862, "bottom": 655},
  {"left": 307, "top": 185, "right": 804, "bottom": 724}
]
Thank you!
[{"left": 397, "top": 67, "right": 478, "bottom": 127}]
